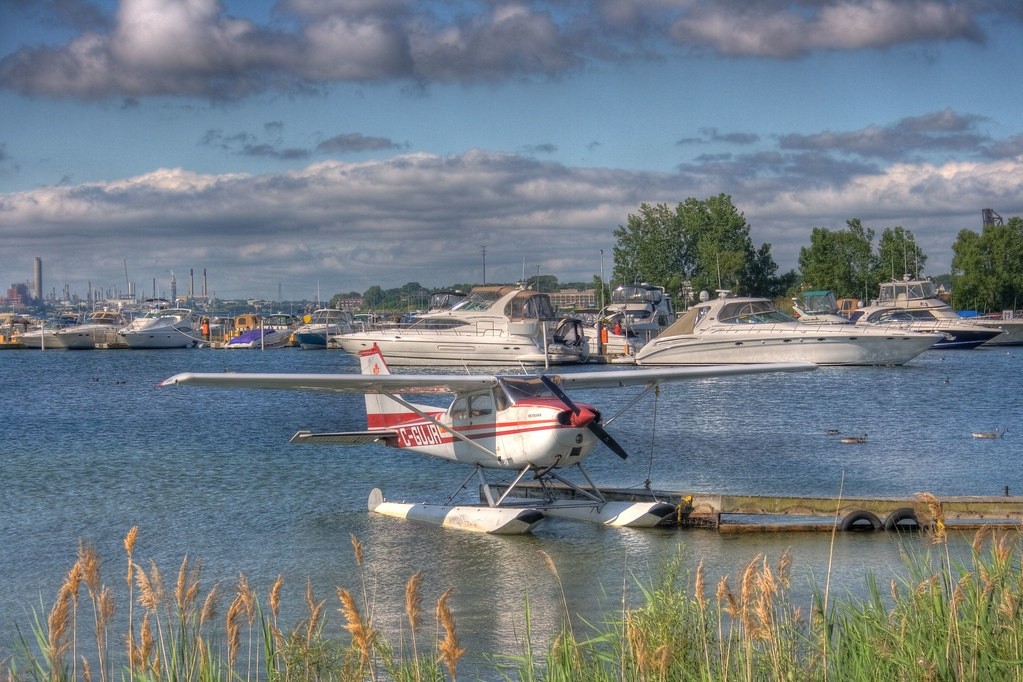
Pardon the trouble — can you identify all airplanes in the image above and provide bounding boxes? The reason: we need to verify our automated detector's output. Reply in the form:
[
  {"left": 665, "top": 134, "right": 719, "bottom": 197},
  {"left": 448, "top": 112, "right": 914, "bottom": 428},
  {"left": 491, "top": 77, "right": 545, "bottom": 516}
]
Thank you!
[{"left": 157, "top": 342, "right": 818, "bottom": 540}]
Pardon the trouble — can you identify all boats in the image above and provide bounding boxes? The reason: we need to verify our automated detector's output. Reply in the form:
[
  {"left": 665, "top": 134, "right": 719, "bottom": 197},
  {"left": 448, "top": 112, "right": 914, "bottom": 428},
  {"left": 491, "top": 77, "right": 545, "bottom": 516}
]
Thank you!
[
  {"left": 792, "top": 286, "right": 851, "bottom": 325},
  {"left": 582, "top": 284, "right": 675, "bottom": 356},
  {"left": 612, "top": 289, "right": 941, "bottom": 367},
  {"left": 964, "top": 310, "right": 1023, "bottom": 346},
  {"left": 0, "top": 307, "right": 295, "bottom": 348},
  {"left": 335, "top": 285, "right": 591, "bottom": 366},
  {"left": 293, "top": 289, "right": 467, "bottom": 350},
  {"left": 846, "top": 233, "right": 1003, "bottom": 349}
]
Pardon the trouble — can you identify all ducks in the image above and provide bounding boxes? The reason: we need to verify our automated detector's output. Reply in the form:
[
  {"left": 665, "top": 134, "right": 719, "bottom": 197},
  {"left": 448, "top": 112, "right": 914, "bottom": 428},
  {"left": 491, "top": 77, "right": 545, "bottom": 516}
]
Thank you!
[
  {"left": 1005, "top": 350, "right": 1010, "bottom": 355},
  {"left": 943, "top": 376, "right": 952, "bottom": 384},
  {"left": 939, "top": 354, "right": 945, "bottom": 360},
  {"left": 836, "top": 433, "right": 869, "bottom": 444},
  {"left": 971, "top": 428, "right": 1009, "bottom": 439},
  {"left": 824, "top": 429, "right": 841, "bottom": 435}
]
